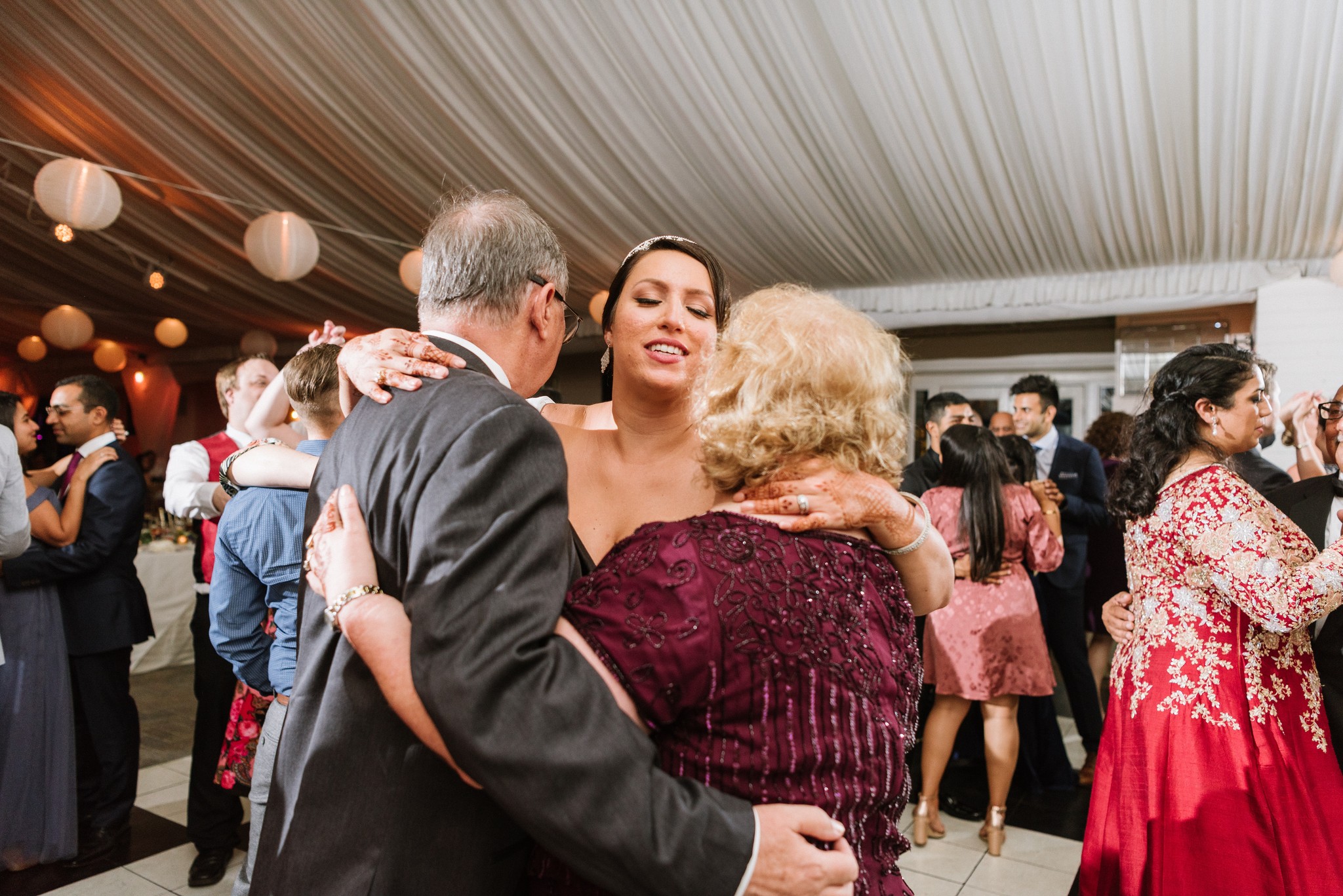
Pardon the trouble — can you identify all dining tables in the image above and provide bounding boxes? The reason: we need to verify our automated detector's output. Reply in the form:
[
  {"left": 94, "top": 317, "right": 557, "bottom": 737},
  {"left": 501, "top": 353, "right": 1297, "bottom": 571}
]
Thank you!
[{"left": 122, "top": 535, "right": 203, "bottom": 673}]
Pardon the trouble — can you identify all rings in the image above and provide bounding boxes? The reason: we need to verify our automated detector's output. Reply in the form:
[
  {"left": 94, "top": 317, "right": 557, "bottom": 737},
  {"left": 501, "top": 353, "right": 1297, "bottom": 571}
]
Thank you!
[
  {"left": 303, "top": 552, "right": 314, "bottom": 571},
  {"left": 374, "top": 368, "right": 387, "bottom": 385},
  {"left": 796, "top": 494, "right": 809, "bottom": 515},
  {"left": 305, "top": 532, "right": 316, "bottom": 549}
]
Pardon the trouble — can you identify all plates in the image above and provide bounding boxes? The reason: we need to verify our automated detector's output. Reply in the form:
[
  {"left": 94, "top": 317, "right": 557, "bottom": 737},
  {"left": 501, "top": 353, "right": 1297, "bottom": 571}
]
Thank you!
[{"left": 149, "top": 539, "right": 176, "bottom": 551}]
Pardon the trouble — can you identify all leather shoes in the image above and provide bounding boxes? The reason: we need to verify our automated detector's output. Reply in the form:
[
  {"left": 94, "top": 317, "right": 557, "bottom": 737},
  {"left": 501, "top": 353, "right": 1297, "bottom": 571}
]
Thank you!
[
  {"left": 189, "top": 839, "right": 238, "bottom": 886},
  {"left": 1076, "top": 754, "right": 1101, "bottom": 787},
  {"left": 67, "top": 828, "right": 135, "bottom": 868},
  {"left": 942, "top": 790, "right": 983, "bottom": 820}
]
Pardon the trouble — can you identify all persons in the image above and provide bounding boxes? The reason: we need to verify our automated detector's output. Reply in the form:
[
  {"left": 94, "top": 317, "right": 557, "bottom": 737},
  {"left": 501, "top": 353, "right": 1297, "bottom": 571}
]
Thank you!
[
  {"left": 243, "top": 191, "right": 859, "bottom": 896},
  {"left": 302, "top": 278, "right": 923, "bottom": 895},
  {"left": 1, "top": 376, "right": 155, "bottom": 872},
  {"left": 157, "top": 322, "right": 348, "bottom": 888},
  {"left": 338, "top": 235, "right": 953, "bottom": 618},
  {"left": 895, "top": 340, "right": 1343, "bottom": 895}
]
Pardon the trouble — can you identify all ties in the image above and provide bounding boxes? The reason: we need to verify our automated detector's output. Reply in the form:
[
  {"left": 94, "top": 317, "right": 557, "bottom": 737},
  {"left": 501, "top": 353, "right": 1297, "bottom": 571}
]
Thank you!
[{"left": 57, "top": 451, "right": 83, "bottom": 502}]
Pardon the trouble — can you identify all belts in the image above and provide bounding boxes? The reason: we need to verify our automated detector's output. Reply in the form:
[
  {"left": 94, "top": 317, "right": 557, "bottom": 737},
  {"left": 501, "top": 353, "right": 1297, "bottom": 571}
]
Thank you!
[{"left": 275, "top": 692, "right": 290, "bottom": 706}]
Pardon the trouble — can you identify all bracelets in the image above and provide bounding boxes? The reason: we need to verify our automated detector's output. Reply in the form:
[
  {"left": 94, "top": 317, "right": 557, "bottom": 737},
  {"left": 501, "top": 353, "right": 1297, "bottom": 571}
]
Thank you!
[{"left": 885, "top": 491, "right": 930, "bottom": 555}]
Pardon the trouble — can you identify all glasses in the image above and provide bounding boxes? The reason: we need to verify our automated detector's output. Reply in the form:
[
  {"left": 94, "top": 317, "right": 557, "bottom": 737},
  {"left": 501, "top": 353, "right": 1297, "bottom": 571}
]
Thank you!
[
  {"left": 527, "top": 274, "right": 583, "bottom": 346},
  {"left": 46, "top": 404, "right": 83, "bottom": 417}
]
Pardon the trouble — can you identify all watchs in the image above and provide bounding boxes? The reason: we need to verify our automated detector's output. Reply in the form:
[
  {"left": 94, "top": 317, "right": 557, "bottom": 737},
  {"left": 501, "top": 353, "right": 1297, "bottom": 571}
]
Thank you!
[{"left": 324, "top": 585, "right": 383, "bottom": 632}]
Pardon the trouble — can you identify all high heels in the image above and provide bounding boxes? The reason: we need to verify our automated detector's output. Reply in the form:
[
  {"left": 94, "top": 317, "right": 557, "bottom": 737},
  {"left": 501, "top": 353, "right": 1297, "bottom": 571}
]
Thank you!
[
  {"left": 980, "top": 805, "right": 1007, "bottom": 857},
  {"left": 915, "top": 794, "right": 944, "bottom": 844}
]
